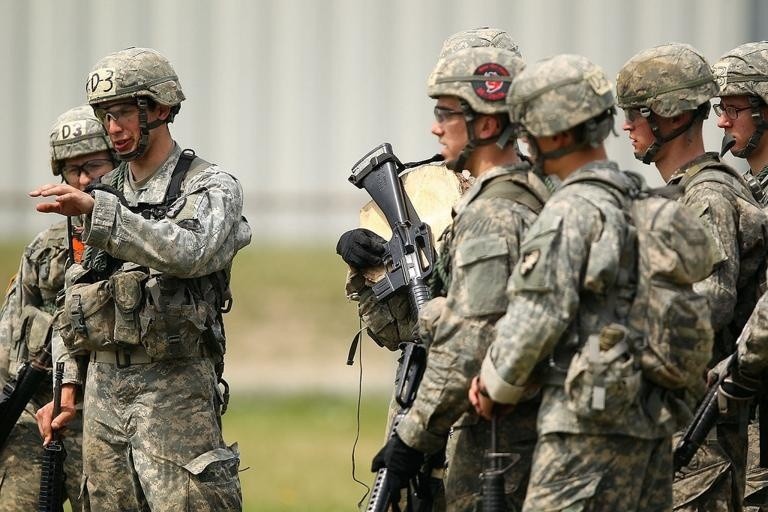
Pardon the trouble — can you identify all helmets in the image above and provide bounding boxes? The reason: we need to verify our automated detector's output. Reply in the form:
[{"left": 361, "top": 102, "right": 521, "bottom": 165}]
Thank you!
[
  {"left": 439, "top": 26, "right": 521, "bottom": 54},
  {"left": 712, "top": 43, "right": 764, "bottom": 96},
  {"left": 50, "top": 107, "right": 112, "bottom": 176},
  {"left": 84, "top": 46, "right": 185, "bottom": 106},
  {"left": 617, "top": 45, "right": 718, "bottom": 116},
  {"left": 511, "top": 57, "right": 619, "bottom": 140},
  {"left": 428, "top": 51, "right": 517, "bottom": 111}
]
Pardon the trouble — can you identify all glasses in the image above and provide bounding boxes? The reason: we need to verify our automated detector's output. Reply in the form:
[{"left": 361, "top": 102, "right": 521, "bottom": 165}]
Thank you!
[
  {"left": 626, "top": 110, "right": 655, "bottom": 122},
  {"left": 437, "top": 107, "right": 470, "bottom": 123},
  {"left": 715, "top": 105, "right": 762, "bottom": 120},
  {"left": 91, "top": 103, "right": 141, "bottom": 122},
  {"left": 64, "top": 158, "right": 112, "bottom": 187}
]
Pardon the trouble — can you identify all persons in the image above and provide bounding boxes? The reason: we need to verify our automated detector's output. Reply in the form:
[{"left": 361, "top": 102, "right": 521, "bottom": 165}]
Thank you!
[
  {"left": 40, "top": 362, "right": 64, "bottom": 512},
  {"left": 710, "top": 41, "right": 768, "bottom": 216},
  {"left": 708, "top": 289, "right": 767, "bottom": 427},
  {"left": 470, "top": 53, "right": 673, "bottom": 511},
  {"left": 0, "top": 108, "right": 120, "bottom": 512},
  {"left": 29, "top": 47, "right": 250, "bottom": 512},
  {"left": 372, "top": 47, "right": 550, "bottom": 512},
  {"left": 617, "top": 43, "right": 748, "bottom": 507},
  {"left": 337, "top": 28, "right": 546, "bottom": 348}
]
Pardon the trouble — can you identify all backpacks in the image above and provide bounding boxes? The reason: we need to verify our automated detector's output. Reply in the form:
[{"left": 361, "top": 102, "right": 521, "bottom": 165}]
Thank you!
[{"left": 560, "top": 172, "right": 714, "bottom": 392}]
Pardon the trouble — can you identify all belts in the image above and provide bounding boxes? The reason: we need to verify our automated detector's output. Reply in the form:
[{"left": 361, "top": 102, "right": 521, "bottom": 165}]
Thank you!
[{"left": 88, "top": 343, "right": 169, "bottom": 368}]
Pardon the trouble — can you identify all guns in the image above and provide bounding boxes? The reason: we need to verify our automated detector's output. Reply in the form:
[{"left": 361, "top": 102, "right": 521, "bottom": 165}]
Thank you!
[
  {"left": 475, "top": 408, "right": 519, "bottom": 512},
  {"left": 364, "top": 341, "right": 427, "bottom": 512},
  {"left": 671, "top": 379, "right": 716, "bottom": 474},
  {"left": 351, "top": 142, "right": 436, "bottom": 314}
]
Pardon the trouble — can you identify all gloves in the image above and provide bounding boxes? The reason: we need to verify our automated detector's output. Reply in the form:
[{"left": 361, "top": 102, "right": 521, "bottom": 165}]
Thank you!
[
  {"left": 710, "top": 357, "right": 761, "bottom": 425},
  {"left": 337, "top": 229, "right": 389, "bottom": 273},
  {"left": 368, "top": 436, "right": 426, "bottom": 494}
]
